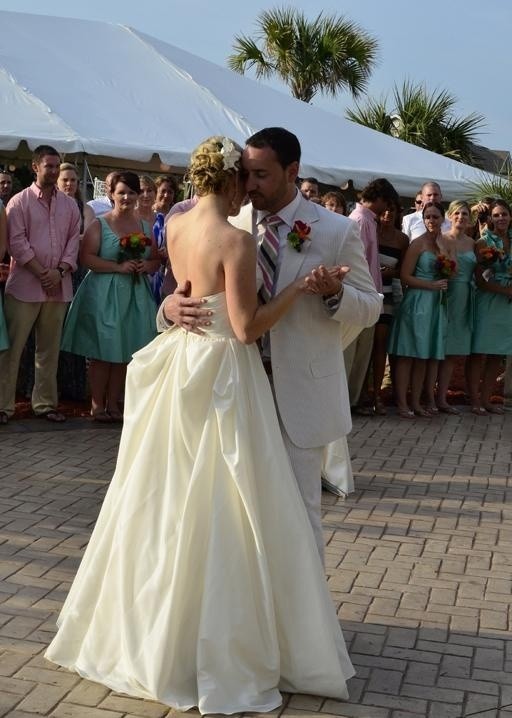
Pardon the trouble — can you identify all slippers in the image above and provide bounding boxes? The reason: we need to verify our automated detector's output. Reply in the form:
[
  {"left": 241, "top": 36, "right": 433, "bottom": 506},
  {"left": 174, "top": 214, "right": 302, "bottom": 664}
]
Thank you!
[
  {"left": 41, "top": 410, "right": 68, "bottom": 423},
  {"left": 0, "top": 412, "right": 9, "bottom": 425}
]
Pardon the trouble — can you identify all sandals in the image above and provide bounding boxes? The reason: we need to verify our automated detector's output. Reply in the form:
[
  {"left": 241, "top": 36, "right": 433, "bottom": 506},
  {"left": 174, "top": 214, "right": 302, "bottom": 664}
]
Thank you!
[
  {"left": 411, "top": 408, "right": 431, "bottom": 417},
  {"left": 435, "top": 402, "right": 461, "bottom": 414},
  {"left": 470, "top": 408, "right": 488, "bottom": 416},
  {"left": 397, "top": 407, "right": 416, "bottom": 419},
  {"left": 106, "top": 410, "right": 123, "bottom": 422},
  {"left": 483, "top": 406, "right": 504, "bottom": 415},
  {"left": 424, "top": 407, "right": 440, "bottom": 416},
  {"left": 90, "top": 409, "right": 114, "bottom": 423}
]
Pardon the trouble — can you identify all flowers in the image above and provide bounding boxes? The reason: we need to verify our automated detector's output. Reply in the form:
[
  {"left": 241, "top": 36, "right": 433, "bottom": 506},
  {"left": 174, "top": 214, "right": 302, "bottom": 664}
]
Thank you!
[
  {"left": 477, "top": 247, "right": 505, "bottom": 285},
  {"left": 431, "top": 255, "right": 457, "bottom": 304},
  {"left": 112, "top": 232, "right": 153, "bottom": 286}
]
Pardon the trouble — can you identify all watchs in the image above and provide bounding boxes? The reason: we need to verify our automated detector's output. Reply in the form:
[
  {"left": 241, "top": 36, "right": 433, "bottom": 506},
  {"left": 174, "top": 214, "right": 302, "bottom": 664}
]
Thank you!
[
  {"left": 56, "top": 267, "right": 66, "bottom": 277},
  {"left": 321, "top": 283, "right": 343, "bottom": 309}
]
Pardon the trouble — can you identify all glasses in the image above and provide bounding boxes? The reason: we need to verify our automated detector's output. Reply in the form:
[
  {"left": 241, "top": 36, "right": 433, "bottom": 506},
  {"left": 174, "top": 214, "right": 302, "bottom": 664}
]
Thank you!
[{"left": 415, "top": 200, "right": 422, "bottom": 204}]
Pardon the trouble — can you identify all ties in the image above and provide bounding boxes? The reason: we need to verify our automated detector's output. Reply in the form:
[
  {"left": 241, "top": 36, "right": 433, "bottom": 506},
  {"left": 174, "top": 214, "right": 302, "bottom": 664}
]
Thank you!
[{"left": 255, "top": 216, "right": 285, "bottom": 354}]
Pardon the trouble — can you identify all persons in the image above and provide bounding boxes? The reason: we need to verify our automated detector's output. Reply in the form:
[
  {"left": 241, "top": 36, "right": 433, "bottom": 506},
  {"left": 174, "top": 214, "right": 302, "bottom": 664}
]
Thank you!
[
  {"left": 359, "top": 196, "right": 410, "bottom": 416},
  {"left": 386, "top": 203, "right": 456, "bottom": 419},
  {"left": 60, "top": 171, "right": 163, "bottom": 422},
  {"left": 0, "top": 198, "right": 10, "bottom": 352},
  {"left": 0, "top": 172, "right": 12, "bottom": 207},
  {"left": 157, "top": 128, "right": 381, "bottom": 575},
  {"left": 465, "top": 199, "right": 512, "bottom": 413},
  {"left": 341, "top": 179, "right": 399, "bottom": 416},
  {"left": 298, "top": 178, "right": 346, "bottom": 217},
  {"left": 56, "top": 163, "right": 89, "bottom": 296},
  {"left": 155, "top": 175, "right": 178, "bottom": 215},
  {"left": 43, "top": 136, "right": 356, "bottom": 715},
  {"left": 425, "top": 199, "right": 477, "bottom": 415},
  {"left": 135, "top": 175, "right": 165, "bottom": 308},
  {"left": 0, "top": 145, "right": 81, "bottom": 426},
  {"left": 402, "top": 182, "right": 496, "bottom": 241}
]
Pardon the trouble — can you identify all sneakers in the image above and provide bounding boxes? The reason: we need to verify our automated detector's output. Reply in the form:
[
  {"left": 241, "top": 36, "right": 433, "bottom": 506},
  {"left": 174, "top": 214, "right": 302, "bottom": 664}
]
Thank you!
[
  {"left": 356, "top": 398, "right": 371, "bottom": 415},
  {"left": 373, "top": 396, "right": 387, "bottom": 415}
]
